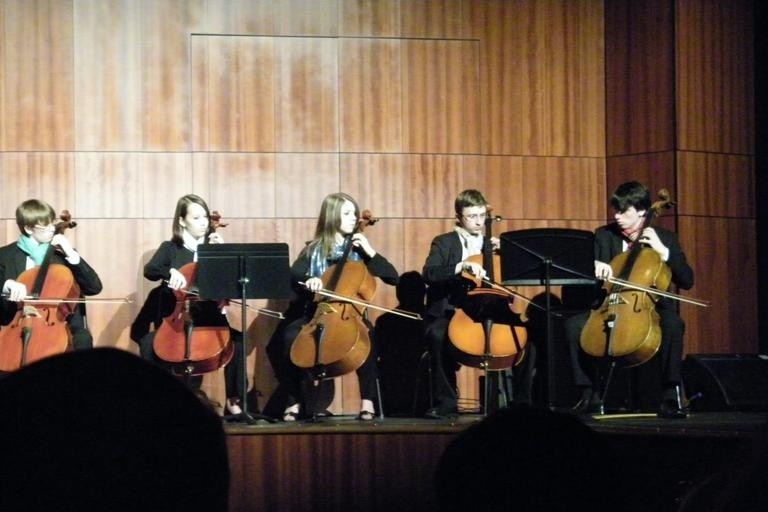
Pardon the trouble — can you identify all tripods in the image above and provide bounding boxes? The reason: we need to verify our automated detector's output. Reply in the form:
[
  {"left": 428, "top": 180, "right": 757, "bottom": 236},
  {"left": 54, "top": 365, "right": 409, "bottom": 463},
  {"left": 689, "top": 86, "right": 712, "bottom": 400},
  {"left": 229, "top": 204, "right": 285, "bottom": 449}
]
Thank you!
[{"left": 217, "top": 294, "right": 280, "bottom": 426}]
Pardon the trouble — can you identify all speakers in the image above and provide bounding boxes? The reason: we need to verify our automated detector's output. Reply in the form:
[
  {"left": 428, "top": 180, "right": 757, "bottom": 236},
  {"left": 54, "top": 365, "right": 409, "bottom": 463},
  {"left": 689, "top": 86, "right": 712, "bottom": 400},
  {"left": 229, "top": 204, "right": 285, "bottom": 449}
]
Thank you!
[{"left": 683, "top": 352, "right": 768, "bottom": 412}]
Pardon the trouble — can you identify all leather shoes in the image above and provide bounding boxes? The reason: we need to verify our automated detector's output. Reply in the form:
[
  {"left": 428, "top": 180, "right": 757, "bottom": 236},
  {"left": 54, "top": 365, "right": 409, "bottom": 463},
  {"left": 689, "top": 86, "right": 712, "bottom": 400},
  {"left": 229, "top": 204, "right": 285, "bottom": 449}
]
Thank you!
[
  {"left": 569, "top": 399, "right": 606, "bottom": 413},
  {"left": 427, "top": 406, "right": 459, "bottom": 420},
  {"left": 657, "top": 400, "right": 686, "bottom": 418}
]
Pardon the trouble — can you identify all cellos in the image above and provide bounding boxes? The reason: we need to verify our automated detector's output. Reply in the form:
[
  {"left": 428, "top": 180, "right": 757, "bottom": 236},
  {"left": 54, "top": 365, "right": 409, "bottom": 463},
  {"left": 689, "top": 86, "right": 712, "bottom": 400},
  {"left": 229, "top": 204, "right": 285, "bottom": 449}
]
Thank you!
[
  {"left": 448, "top": 205, "right": 528, "bottom": 371},
  {"left": 579, "top": 189, "right": 677, "bottom": 370},
  {"left": 290, "top": 210, "right": 379, "bottom": 381},
  {"left": 152, "top": 211, "right": 235, "bottom": 375},
  {"left": 1, "top": 210, "right": 80, "bottom": 372}
]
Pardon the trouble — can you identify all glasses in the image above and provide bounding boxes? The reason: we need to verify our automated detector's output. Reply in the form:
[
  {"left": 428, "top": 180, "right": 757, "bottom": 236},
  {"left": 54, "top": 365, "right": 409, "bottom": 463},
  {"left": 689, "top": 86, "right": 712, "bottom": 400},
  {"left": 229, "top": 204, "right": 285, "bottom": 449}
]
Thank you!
[{"left": 28, "top": 220, "right": 58, "bottom": 232}]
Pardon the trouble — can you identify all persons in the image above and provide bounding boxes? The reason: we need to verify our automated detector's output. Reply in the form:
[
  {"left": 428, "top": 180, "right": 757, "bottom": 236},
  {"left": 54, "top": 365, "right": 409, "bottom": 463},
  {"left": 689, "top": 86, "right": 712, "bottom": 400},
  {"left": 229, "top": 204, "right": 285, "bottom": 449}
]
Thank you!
[
  {"left": 567, "top": 180, "right": 695, "bottom": 416},
  {"left": 0, "top": 345, "right": 231, "bottom": 511},
  {"left": 0, "top": 198, "right": 103, "bottom": 359},
  {"left": 262, "top": 192, "right": 398, "bottom": 422},
  {"left": 417, "top": 188, "right": 537, "bottom": 416},
  {"left": 431, "top": 402, "right": 631, "bottom": 510},
  {"left": 135, "top": 193, "right": 251, "bottom": 423}
]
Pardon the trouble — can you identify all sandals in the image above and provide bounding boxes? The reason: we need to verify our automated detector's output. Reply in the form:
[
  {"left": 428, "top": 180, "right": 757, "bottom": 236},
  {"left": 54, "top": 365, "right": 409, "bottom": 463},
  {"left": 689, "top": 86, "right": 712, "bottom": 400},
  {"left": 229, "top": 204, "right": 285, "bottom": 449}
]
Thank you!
[
  {"left": 223, "top": 406, "right": 255, "bottom": 424},
  {"left": 358, "top": 411, "right": 376, "bottom": 421},
  {"left": 284, "top": 412, "right": 300, "bottom": 422}
]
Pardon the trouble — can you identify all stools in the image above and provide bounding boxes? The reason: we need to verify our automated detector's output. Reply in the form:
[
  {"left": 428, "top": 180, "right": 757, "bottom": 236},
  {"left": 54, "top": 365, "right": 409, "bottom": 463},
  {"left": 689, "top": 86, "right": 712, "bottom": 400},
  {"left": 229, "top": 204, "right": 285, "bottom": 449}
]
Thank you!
[{"left": 409, "top": 345, "right": 512, "bottom": 419}]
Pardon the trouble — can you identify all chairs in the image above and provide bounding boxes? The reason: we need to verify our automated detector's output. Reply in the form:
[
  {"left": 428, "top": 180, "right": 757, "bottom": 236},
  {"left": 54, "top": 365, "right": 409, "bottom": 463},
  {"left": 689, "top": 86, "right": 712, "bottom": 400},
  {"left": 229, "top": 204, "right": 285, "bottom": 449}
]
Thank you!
[
  {"left": 303, "top": 239, "right": 387, "bottom": 422},
  {"left": 57, "top": 243, "right": 90, "bottom": 333},
  {"left": 153, "top": 281, "right": 176, "bottom": 332}
]
